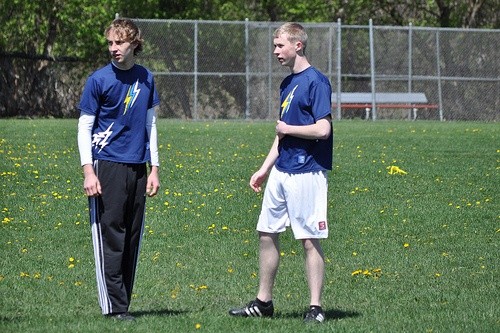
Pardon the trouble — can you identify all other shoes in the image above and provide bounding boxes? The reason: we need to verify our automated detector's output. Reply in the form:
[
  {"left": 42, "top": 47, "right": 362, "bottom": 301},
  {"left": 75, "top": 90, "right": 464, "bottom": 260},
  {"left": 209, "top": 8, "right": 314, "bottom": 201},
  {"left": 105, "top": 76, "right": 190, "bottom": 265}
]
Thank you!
[{"left": 110, "top": 312, "right": 136, "bottom": 322}]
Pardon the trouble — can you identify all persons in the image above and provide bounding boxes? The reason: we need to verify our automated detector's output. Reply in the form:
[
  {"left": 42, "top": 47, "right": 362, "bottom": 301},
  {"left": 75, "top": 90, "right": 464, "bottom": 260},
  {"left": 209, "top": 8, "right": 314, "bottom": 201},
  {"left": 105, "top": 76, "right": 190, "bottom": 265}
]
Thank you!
[
  {"left": 76, "top": 20, "right": 160, "bottom": 322},
  {"left": 228, "top": 22, "right": 333, "bottom": 327}
]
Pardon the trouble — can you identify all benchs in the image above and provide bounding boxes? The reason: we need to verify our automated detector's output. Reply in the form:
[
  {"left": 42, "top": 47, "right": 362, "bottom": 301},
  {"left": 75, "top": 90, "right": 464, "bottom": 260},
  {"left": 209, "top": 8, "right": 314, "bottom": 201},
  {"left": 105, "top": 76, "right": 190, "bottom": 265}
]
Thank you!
[{"left": 331, "top": 92, "right": 439, "bottom": 120}]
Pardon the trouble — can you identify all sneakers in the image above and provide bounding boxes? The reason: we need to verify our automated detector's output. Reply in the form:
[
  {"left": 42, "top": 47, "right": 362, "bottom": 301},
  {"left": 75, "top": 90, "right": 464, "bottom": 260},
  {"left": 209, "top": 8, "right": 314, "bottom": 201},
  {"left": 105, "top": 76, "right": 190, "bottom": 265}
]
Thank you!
[
  {"left": 304, "top": 308, "right": 326, "bottom": 323},
  {"left": 229, "top": 300, "right": 274, "bottom": 318}
]
